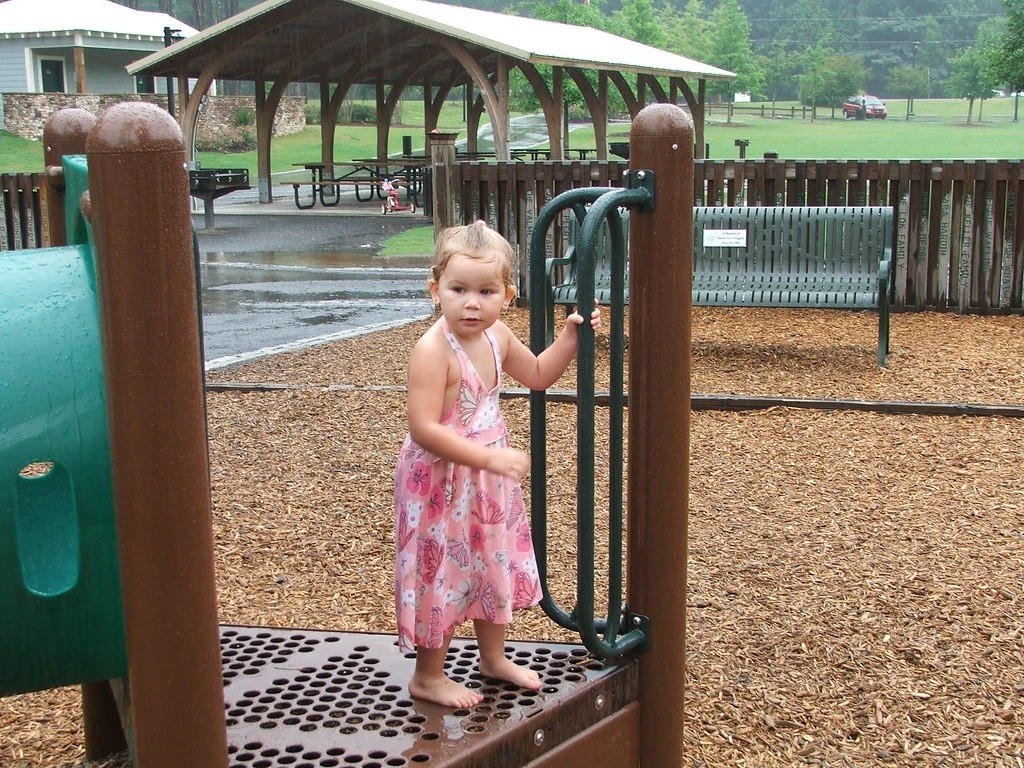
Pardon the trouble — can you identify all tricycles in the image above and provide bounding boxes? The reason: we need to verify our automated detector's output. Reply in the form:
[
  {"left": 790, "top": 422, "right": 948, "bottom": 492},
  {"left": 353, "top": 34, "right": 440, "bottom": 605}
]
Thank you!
[{"left": 371, "top": 178, "right": 417, "bottom": 214}]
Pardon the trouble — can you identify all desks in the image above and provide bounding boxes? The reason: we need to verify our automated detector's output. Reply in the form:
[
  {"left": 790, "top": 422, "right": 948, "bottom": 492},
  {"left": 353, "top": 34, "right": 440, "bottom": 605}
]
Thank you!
[
  {"left": 510, "top": 148, "right": 597, "bottom": 160},
  {"left": 458, "top": 151, "right": 551, "bottom": 160},
  {"left": 352, "top": 157, "right": 485, "bottom": 201},
  {"left": 292, "top": 162, "right": 430, "bottom": 207},
  {"left": 402, "top": 154, "right": 526, "bottom": 160}
]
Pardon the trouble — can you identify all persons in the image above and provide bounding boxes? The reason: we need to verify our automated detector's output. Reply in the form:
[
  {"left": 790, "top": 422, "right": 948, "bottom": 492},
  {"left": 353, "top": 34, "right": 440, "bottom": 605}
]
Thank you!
[{"left": 392, "top": 221, "right": 601, "bottom": 707}]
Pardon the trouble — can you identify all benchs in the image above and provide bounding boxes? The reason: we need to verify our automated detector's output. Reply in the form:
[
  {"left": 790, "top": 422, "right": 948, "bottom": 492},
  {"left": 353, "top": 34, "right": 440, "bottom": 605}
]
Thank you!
[
  {"left": 279, "top": 173, "right": 424, "bottom": 214},
  {"left": 545, "top": 206, "right": 895, "bottom": 367}
]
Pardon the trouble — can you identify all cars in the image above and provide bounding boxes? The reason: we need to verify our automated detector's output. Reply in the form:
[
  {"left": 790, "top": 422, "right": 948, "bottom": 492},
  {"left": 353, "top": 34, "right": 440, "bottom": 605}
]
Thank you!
[{"left": 843, "top": 95, "right": 887, "bottom": 119}]
